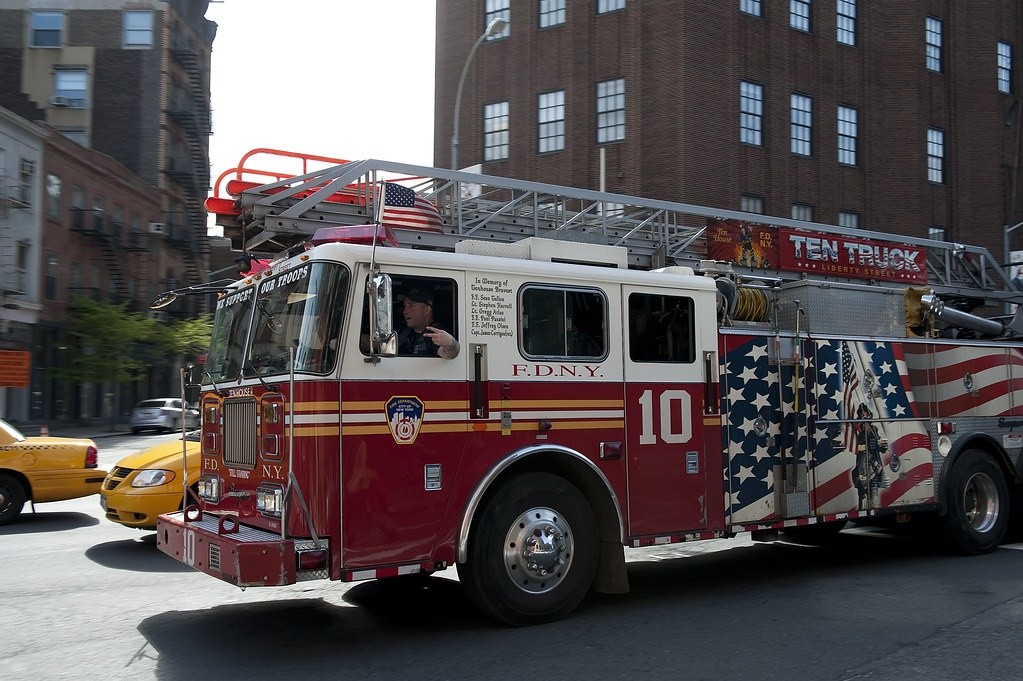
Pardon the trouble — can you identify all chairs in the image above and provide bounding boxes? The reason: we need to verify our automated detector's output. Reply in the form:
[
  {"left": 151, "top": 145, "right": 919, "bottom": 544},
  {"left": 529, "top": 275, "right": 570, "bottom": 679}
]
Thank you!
[{"left": 433, "top": 288, "right": 453, "bottom": 330}]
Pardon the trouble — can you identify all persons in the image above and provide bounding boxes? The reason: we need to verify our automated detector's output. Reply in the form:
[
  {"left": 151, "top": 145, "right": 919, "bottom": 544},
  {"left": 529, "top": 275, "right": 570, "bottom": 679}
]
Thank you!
[
  {"left": 293, "top": 315, "right": 339, "bottom": 364},
  {"left": 361, "top": 287, "right": 460, "bottom": 360}
]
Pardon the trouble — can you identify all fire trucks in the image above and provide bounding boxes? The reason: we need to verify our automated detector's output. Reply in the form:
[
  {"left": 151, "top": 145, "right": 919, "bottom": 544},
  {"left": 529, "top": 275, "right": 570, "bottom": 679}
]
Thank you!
[{"left": 158, "top": 145, "right": 1023, "bottom": 622}]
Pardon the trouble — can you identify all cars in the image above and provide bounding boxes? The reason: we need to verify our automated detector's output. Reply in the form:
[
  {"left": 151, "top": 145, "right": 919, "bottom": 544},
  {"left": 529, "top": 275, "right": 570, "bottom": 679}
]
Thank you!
[
  {"left": 131, "top": 396, "right": 202, "bottom": 434},
  {"left": 0, "top": 418, "right": 111, "bottom": 525},
  {"left": 99, "top": 427, "right": 203, "bottom": 531}
]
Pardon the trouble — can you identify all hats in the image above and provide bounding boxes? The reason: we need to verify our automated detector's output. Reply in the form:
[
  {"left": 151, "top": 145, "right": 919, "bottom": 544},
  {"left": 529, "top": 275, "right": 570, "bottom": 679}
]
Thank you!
[{"left": 405, "top": 288, "right": 432, "bottom": 307}]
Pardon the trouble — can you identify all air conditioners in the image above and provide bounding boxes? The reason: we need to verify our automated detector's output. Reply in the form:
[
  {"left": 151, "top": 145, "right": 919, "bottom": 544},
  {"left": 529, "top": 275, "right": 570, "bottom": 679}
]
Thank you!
[{"left": 153, "top": 223, "right": 170, "bottom": 236}]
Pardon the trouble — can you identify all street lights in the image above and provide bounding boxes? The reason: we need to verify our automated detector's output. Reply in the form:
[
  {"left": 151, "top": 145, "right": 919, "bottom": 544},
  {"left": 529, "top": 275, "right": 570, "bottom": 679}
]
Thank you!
[{"left": 449, "top": 15, "right": 508, "bottom": 221}]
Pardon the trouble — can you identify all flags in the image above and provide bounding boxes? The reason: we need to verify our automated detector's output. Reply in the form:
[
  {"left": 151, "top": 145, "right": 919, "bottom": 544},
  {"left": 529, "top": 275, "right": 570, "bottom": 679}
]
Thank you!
[{"left": 375, "top": 177, "right": 444, "bottom": 235}]
